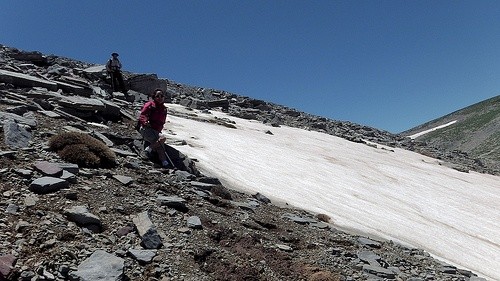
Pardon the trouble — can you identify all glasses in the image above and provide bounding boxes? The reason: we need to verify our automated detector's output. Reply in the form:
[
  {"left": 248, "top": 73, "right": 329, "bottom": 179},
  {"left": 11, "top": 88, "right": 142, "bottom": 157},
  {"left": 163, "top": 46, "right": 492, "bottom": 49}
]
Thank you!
[{"left": 155, "top": 95, "right": 164, "bottom": 98}]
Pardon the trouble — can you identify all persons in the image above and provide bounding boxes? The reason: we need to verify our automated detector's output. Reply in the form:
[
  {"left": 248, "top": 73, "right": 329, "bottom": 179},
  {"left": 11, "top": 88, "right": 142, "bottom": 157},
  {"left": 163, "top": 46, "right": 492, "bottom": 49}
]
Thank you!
[
  {"left": 106, "top": 53, "right": 125, "bottom": 92},
  {"left": 141, "top": 89, "right": 167, "bottom": 165}
]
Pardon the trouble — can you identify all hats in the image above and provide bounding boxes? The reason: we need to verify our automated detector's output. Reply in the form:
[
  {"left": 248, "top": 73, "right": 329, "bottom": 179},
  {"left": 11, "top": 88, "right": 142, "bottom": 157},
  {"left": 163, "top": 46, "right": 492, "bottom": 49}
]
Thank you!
[{"left": 112, "top": 53, "right": 119, "bottom": 56}]
